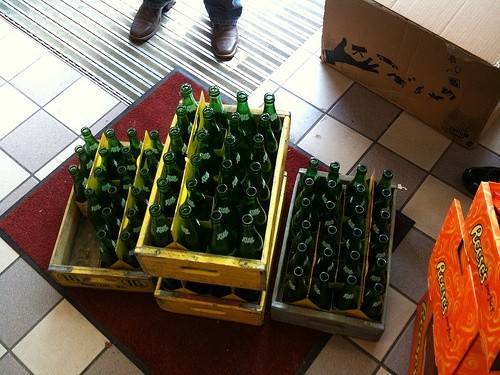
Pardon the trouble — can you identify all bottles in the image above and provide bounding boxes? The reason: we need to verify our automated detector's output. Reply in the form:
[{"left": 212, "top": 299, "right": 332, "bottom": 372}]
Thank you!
[
  {"left": 280, "top": 156, "right": 394, "bottom": 322},
  {"left": 67, "top": 83, "right": 282, "bottom": 305}
]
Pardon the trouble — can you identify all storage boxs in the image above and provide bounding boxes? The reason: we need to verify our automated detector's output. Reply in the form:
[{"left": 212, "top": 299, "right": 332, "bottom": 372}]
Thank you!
[
  {"left": 270, "top": 169, "right": 400, "bottom": 342},
  {"left": 321, "top": 0, "right": 499, "bottom": 150},
  {"left": 135, "top": 100, "right": 290, "bottom": 290},
  {"left": 48, "top": 140, "right": 157, "bottom": 292},
  {"left": 154, "top": 171, "right": 289, "bottom": 325},
  {"left": 410, "top": 181, "right": 500, "bottom": 375}
]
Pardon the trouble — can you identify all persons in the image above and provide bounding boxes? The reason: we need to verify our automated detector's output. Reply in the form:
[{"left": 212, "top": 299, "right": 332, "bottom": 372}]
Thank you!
[{"left": 129, "top": 0, "right": 242, "bottom": 59}]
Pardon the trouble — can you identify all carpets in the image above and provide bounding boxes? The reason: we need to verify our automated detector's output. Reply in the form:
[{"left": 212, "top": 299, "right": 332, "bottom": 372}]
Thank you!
[{"left": 0, "top": 67, "right": 418, "bottom": 375}]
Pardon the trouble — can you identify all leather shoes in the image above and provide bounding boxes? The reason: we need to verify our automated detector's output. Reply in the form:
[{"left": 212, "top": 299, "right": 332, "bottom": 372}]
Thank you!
[
  {"left": 211, "top": 19, "right": 239, "bottom": 59},
  {"left": 129, "top": 0, "right": 177, "bottom": 41}
]
